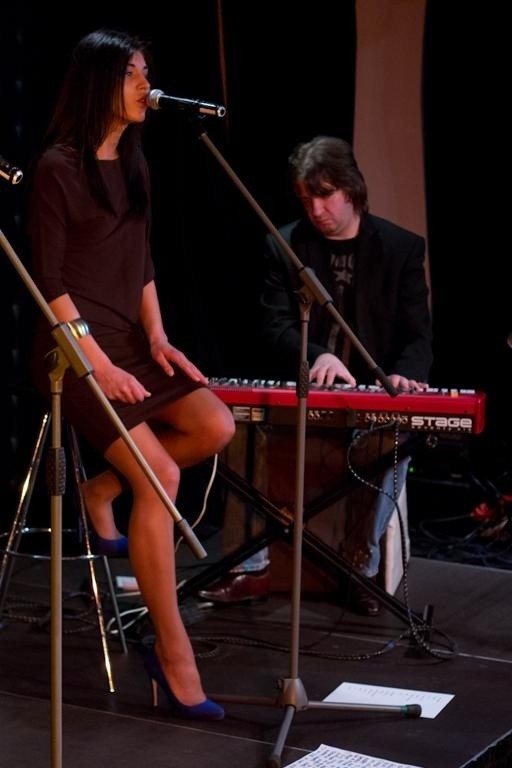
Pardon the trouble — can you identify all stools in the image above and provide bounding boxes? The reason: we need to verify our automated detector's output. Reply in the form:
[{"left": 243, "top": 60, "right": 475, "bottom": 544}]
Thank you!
[{"left": 222, "top": 420, "right": 413, "bottom": 604}]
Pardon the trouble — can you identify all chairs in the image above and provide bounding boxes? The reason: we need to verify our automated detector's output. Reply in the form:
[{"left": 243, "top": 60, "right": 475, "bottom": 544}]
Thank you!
[{"left": 0, "top": 402, "right": 150, "bottom": 656}]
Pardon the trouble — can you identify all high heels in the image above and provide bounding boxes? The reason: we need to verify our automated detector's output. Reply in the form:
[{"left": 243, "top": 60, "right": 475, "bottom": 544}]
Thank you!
[
  {"left": 73, "top": 485, "right": 128, "bottom": 556},
  {"left": 148, "top": 650, "right": 224, "bottom": 721}
]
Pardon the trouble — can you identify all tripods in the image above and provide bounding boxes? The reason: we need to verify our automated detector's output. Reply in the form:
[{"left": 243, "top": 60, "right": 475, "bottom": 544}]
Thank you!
[{"left": 190, "top": 118, "right": 422, "bottom": 768}]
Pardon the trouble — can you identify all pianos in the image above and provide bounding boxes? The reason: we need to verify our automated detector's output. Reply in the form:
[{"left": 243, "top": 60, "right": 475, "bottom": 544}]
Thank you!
[{"left": 196, "top": 377, "right": 487, "bottom": 440}]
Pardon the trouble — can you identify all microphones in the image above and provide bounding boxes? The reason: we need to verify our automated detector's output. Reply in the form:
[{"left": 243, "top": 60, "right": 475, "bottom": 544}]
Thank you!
[
  {"left": 147, "top": 89, "right": 226, "bottom": 118},
  {"left": 0, "top": 158, "right": 24, "bottom": 184}
]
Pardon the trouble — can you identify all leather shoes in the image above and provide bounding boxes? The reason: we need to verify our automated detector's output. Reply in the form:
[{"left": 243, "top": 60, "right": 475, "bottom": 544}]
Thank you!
[
  {"left": 198, "top": 567, "right": 270, "bottom": 603},
  {"left": 339, "top": 573, "right": 379, "bottom": 616}
]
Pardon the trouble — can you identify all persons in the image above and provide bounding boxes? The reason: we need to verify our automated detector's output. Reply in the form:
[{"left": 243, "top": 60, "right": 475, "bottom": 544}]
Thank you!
[
  {"left": 194, "top": 132, "right": 437, "bottom": 619},
  {"left": 22, "top": 26, "right": 240, "bottom": 726}
]
